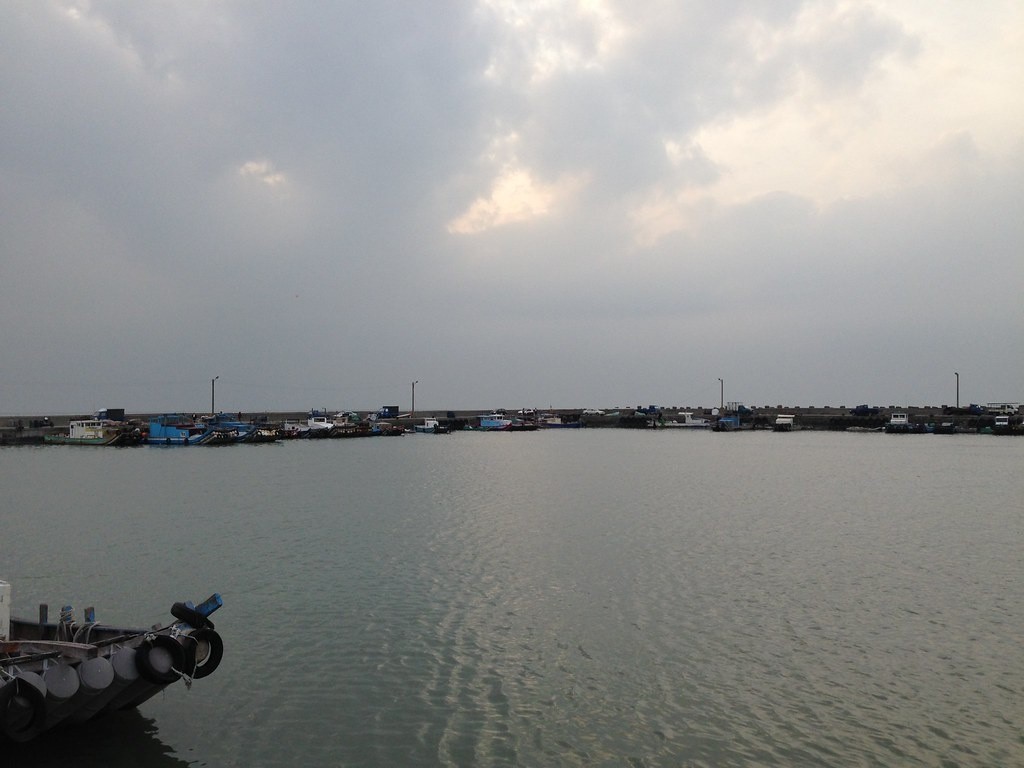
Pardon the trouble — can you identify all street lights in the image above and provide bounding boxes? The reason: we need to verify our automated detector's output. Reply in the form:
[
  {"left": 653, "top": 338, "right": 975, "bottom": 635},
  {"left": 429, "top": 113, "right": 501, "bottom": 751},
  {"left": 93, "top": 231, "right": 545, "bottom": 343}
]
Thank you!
[
  {"left": 411, "top": 380, "right": 419, "bottom": 418},
  {"left": 716, "top": 378, "right": 724, "bottom": 407},
  {"left": 211, "top": 376, "right": 221, "bottom": 416},
  {"left": 953, "top": 371, "right": 960, "bottom": 414}
]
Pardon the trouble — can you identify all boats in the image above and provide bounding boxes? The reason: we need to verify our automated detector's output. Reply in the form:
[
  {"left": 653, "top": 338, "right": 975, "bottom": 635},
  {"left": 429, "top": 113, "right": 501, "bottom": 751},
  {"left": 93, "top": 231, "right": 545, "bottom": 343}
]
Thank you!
[
  {"left": 43, "top": 418, "right": 149, "bottom": 447},
  {"left": 476, "top": 413, "right": 588, "bottom": 431},
  {"left": 0, "top": 576, "right": 225, "bottom": 747},
  {"left": 199, "top": 408, "right": 405, "bottom": 444},
  {"left": 413, "top": 416, "right": 452, "bottom": 434},
  {"left": 890, "top": 412, "right": 909, "bottom": 430},
  {"left": 775, "top": 414, "right": 795, "bottom": 432},
  {"left": 994, "top": 415, "right": 1010, "bottom": 429},
  {"left": 140, "top": 414, "right": 239, "bottom": 445}
]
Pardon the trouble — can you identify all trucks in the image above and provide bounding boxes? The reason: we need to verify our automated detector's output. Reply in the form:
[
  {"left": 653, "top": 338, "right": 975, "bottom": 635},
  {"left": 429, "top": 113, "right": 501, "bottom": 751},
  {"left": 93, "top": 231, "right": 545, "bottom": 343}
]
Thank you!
[
  {"left": 89, "top": 407, "right": 126, "bottom": 422},
  {"left": 377, "top": 405, "right": 400, "bottom": 419}
]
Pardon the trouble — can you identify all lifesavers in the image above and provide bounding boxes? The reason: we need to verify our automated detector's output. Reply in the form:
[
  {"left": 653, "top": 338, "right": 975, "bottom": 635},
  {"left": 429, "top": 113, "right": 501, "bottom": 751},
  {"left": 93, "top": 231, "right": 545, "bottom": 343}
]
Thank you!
[
  {"left": 166, "top": 438, "right": 170, "bottom": 444},
  {"left": 183, "top": 438, "right": 188, "bottom": 446},
  {"left": 184, "top": 628, "right": 224, "bottom": 679},
  {"left": 135, "top": 635, "right": 187, "bottom": 684}
]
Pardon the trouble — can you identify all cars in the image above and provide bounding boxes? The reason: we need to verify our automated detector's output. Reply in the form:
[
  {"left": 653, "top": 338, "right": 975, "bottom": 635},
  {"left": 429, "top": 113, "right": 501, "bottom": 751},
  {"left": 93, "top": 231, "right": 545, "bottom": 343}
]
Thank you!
[
  {"left": 583, "top": 408, "right": 606, "bottom": 416},
  {"left": 518, "top": 409, "right": 534, "bottom": 416},
  {"left": 305, "top": 410, "right": 330, "bottom": 421},
  {"left": 489, "top": 408, "right": 508, "bottom": 416},
  {"left": 332, "top": 411, "right": 356, "bottom": 421}
]
